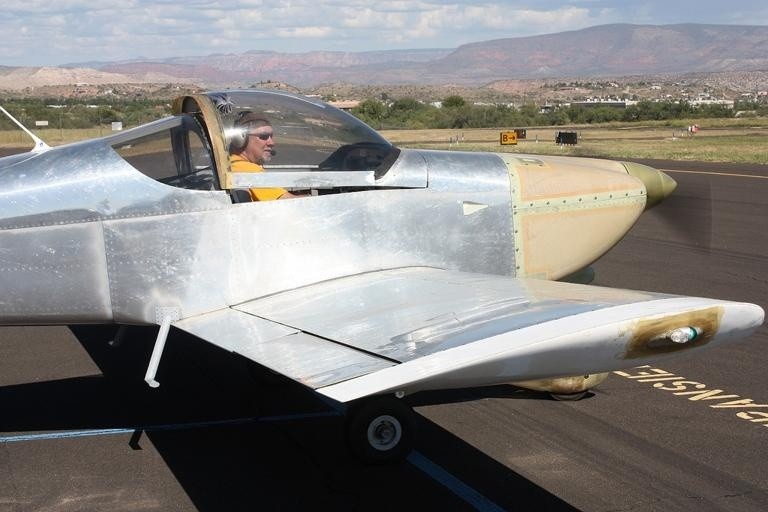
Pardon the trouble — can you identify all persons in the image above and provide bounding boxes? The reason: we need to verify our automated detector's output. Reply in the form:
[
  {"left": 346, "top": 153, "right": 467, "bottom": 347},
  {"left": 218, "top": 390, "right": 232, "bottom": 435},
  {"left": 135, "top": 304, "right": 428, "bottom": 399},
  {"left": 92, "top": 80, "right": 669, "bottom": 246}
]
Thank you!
[
  {"left": 230, "top": 110, "right": 311, "bottom": 201},
  {"left": 687, "top": 124, "right": 698, "bottom": 135}
]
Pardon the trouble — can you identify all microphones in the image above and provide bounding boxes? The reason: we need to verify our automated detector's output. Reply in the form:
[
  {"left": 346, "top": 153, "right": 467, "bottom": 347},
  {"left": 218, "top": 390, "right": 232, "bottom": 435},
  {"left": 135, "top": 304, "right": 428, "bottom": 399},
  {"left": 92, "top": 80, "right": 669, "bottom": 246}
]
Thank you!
[{"left": 271, "top": 150, "right": 276, "bottom": 156}]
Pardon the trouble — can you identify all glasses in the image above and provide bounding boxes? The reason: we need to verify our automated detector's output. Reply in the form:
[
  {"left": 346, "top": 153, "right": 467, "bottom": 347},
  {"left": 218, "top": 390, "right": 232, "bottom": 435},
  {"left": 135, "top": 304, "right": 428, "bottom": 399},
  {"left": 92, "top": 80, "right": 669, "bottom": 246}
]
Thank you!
[{"left": 247, "top": 132, "right": 273, "bottom": 140}]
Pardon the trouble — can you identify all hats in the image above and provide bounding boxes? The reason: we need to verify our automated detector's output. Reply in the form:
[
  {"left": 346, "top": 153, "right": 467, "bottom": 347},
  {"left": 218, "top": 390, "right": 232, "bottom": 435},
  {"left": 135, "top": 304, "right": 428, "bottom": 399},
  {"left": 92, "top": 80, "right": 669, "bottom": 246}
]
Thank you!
[{"left": 234, "top": 110, "right": 272, "bottom": 128}]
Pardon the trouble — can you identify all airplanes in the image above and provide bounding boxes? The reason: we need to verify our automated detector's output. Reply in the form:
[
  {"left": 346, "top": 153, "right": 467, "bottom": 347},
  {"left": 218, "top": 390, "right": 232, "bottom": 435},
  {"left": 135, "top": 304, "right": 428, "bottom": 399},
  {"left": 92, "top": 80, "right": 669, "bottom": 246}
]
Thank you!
[{"left": 0, "top": 88, "right": 763, "bottom": 467}]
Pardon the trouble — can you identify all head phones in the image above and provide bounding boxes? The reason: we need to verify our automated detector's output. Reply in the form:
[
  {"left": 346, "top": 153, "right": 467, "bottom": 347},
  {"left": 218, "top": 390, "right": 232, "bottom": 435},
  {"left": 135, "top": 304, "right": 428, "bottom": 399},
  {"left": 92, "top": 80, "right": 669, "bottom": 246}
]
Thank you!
[{"left": 228, "top": 110, "right": 270, "bottom": 155}]
